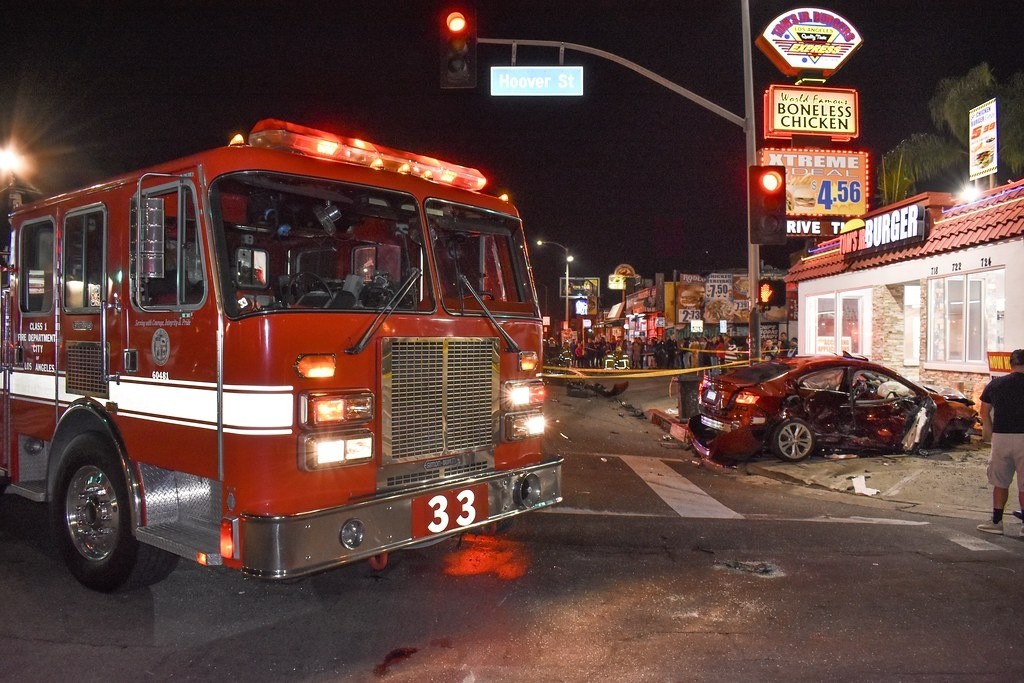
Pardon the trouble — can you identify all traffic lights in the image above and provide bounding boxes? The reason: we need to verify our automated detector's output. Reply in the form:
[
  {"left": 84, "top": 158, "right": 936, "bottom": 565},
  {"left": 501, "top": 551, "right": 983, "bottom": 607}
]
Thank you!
[
  {"left": 437, "top": 7, "right": 480, "bottom": 92},
  {"left": 748, "top": 163, "right": 788, "bottom": 197},
  {"left": 759, "top": 277, "right": 787, "bottom": 307}
]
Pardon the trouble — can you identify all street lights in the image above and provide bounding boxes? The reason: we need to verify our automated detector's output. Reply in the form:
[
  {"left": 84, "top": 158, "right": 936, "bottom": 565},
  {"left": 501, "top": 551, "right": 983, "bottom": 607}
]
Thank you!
[{"left": 532, "top": 234, "right": 574, "bottom": 348}]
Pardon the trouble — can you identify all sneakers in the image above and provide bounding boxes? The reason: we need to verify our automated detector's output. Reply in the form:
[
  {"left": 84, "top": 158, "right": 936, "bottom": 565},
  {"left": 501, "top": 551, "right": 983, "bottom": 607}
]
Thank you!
[
  {"left": 1019, "top": 526, "right": 1024, "bottom": 537},
  {"left": 977, "top": 516, "right": 1003, "bottom": 534}
]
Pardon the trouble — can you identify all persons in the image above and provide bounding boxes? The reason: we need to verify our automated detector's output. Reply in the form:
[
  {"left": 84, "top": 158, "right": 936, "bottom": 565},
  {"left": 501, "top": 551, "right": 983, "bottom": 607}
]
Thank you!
[
  {"left": 569, "top": 332, "right": 798, "bottom": 369},
  {"left": 977, "top": 349, "right": 1024, "bottom": 534}
]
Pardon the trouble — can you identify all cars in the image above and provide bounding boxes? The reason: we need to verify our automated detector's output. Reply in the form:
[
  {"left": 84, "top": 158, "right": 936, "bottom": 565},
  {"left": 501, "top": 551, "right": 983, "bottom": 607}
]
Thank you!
[{"left": 685, "top": 346, "right": 980, "bottom": 463}]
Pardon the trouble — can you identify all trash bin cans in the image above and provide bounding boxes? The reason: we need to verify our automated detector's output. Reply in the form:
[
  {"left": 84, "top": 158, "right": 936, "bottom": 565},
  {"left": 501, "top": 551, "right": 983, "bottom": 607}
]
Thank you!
[{"left": 669, "top": 375, "right": 702, "bottom": 423}]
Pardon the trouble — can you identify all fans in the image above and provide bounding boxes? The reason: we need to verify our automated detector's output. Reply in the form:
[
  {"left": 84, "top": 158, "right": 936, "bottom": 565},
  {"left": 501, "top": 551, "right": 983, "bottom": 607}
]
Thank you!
[{"left": 314, "top": 199, "right": 341, "bottom": 234}]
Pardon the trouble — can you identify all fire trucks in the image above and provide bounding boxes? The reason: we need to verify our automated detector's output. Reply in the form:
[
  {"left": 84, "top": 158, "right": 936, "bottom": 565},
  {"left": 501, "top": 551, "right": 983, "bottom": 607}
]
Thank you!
[{"left": 0, "top": 116, "right": 566, "bottom": 595}]
